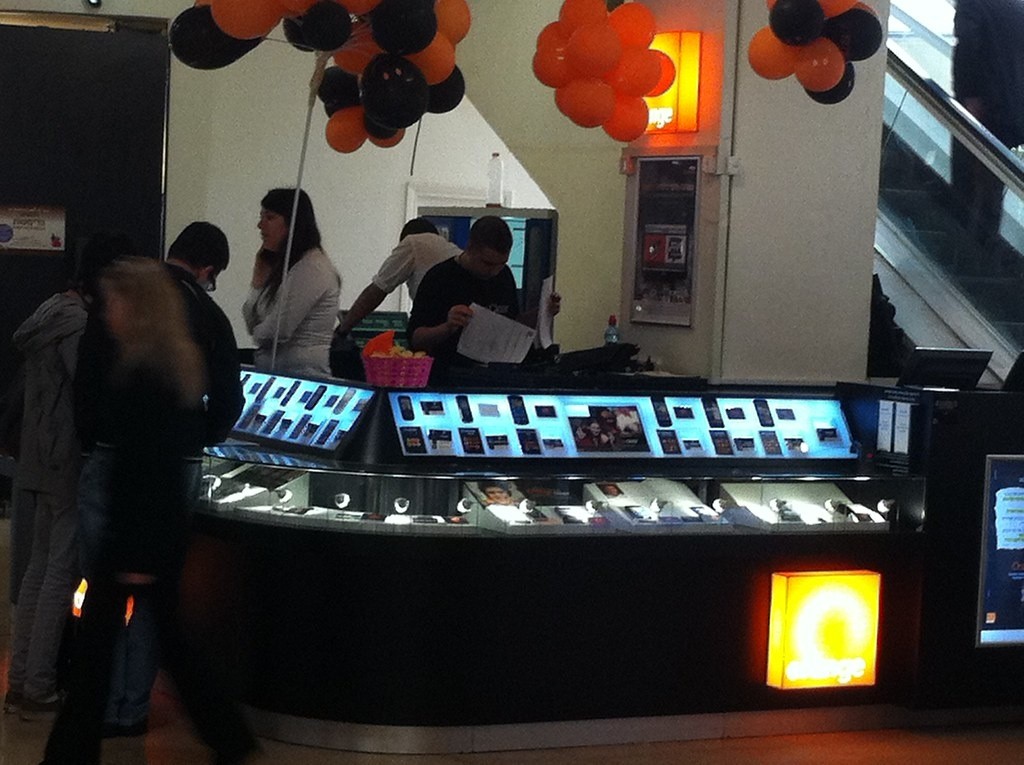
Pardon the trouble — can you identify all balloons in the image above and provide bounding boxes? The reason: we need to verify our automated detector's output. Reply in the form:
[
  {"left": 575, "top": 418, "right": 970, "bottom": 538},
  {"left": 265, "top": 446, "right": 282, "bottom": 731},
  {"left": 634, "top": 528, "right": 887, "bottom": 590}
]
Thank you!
[
  {"left": 166, "top": 0, "right": 473, "bottom": 155},
  {"left": 532, "top": 1, "right": 678, "bottom": 142},
  {"left": 747, "top": 1, "right": 885, "bottom": 105}
]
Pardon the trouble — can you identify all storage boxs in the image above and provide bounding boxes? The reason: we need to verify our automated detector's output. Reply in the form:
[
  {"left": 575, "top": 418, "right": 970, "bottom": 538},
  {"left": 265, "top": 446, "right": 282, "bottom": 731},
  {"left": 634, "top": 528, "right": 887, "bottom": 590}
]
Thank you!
[
  {"left": 199, "top": 463, "right": 310, "bottom": 508},
  {"left": 458, "top": 478, "right": 889, "bottom": 533}
]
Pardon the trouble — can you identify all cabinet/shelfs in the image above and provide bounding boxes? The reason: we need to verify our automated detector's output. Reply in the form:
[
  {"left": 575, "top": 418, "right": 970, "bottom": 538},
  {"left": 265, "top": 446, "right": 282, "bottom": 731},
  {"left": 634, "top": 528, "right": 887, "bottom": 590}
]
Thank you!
[{"left": 149, "top": 446, "right": 931, "bottom": 757}]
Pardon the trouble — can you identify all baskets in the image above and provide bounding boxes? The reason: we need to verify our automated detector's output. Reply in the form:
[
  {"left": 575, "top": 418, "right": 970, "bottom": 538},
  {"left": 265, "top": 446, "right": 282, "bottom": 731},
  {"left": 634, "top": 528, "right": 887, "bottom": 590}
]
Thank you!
[{"left": 363, "top": 355, "right": 433, "bottom": 388}]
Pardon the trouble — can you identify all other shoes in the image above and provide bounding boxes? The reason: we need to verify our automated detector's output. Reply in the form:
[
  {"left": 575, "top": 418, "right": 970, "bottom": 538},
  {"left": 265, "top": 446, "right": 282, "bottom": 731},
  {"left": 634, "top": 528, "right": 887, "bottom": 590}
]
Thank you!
[
  {"left": 20, "top": 692, "right": 66, "bottom": 720},
  {"left": 102, "top": 716, "right": 149, "bottom": 740},
  {"left": 210, "top": 737, "right": 259, "bottom": 765},
  {"left": 3, "top": 690, "right": 26, "bottom": 714}
]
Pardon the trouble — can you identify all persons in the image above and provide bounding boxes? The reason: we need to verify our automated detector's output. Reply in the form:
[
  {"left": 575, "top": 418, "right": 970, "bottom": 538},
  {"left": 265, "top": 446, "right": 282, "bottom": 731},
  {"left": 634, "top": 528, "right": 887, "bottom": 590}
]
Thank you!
[
  {"left": 577, "top": 406, "right": 643, "bottom": 451},
  {"left": 71, "top": 220, "right": 245, "bottom": 736},
  {"left": 601, "top": 481, "right": 623, "bottom": 496},
  {"left": 0, "top": 227, "right": 143, "bottom": 724},
  {"left": 246, "top": 187, "right": 338, "bottom": 377},
  {"left": 38, "top": 254, "right": 265, "bottom": 764},
  {"left": 406, "top": 215, "right": 560, "bottom": 351},
  {"left": 475, "top": 479, "right": 515, "bottom": 506},
  {"left": 336, "top": 217, "right": 464, "bottom": 338}
]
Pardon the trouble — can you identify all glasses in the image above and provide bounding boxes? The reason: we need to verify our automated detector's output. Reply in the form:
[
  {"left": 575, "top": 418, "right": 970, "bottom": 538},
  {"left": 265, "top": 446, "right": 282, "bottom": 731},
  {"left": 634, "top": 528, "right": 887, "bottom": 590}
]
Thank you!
[{"left": 204, "top": 267, "right": 216, "bottom": 292}]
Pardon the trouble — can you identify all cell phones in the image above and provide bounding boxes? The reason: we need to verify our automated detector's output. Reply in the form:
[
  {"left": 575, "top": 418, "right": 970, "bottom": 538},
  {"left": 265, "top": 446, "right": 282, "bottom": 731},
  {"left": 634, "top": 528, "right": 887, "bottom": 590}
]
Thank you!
[
  {"left": 649, "top": 394, "right": 786, "bottom": 457},
  {"left": 396, "top": 393, "right": 541, "bottom": 455},
  {"left": 235, "top": 373, "right": 356, "bottom": 446},
  {"left": 624, "top": 505, "right": 652, "bottom": 519},
  {"left": 690, "top": 506, "right": 709, "bottom": 517}
]
